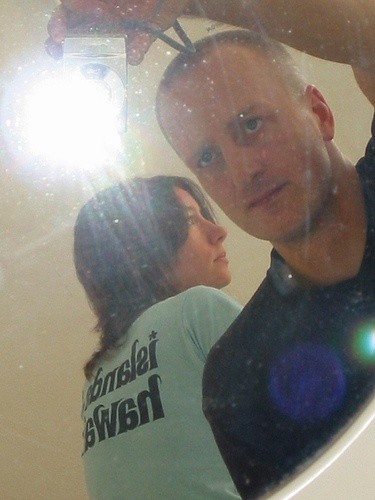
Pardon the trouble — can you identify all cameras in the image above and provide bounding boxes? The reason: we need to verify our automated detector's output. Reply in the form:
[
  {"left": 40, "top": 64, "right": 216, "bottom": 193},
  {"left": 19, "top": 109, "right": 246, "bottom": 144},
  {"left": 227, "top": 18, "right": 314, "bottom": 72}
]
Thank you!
[{"left": 61, "top": 32, "right": 128, "bottom": 133}]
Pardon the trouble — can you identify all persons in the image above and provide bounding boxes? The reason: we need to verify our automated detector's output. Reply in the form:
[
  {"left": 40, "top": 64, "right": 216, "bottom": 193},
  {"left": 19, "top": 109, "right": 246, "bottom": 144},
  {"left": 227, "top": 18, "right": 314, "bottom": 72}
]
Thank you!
[
  {"left": 71, "top": 172, "right": 245, "bottom": 500},
  {"left": 47, "top": 0, "right": 375, "bottom": 500}
]
